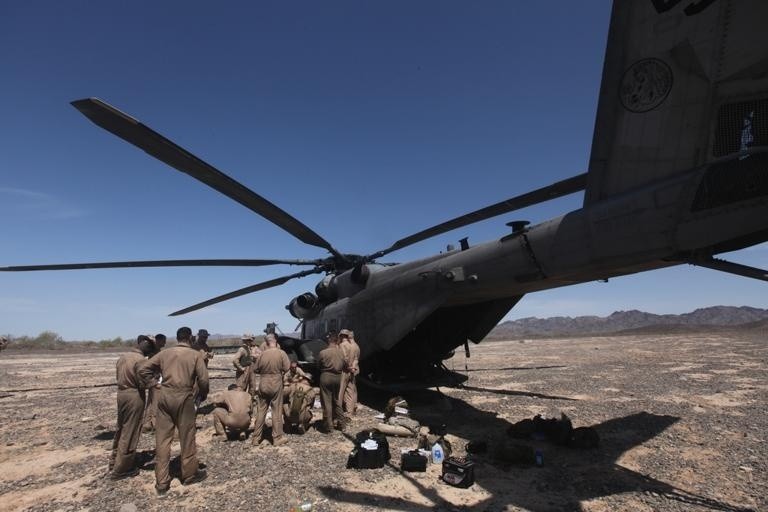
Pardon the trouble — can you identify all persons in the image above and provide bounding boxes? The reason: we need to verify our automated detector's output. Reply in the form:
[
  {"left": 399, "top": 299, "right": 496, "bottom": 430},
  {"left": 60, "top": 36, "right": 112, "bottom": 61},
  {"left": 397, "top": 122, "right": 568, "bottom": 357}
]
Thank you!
[
  {"left": 135, "top": 326, "right": 209, "bottom": 497},
  {"left": 207, "top": 327, "right": 361, "bottom": 447},
  {"left": 189, "top": 328, "right": 214, "bottom": 429},
  {"left": 107, "top": 333, "right": 159, "bottom": 482},
  {"left": 141, "top": 333, "right": 166, "bottom": 430}
]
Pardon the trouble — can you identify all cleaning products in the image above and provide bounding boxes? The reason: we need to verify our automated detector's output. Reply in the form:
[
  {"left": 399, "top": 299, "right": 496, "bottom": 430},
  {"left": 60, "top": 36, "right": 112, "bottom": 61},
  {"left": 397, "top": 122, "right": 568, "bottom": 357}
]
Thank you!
[
  {"left": 432, "top": 442, "right": 443, "bottom": 464},
  {"left": 535, "top": 447, "right": 544, "bottom": 467}
]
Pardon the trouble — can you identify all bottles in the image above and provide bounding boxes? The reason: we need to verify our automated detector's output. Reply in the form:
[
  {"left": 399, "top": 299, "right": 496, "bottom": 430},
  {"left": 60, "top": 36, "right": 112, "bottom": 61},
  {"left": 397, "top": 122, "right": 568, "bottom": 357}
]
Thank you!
[{"left": 536, "top": 446, "right": 543, "bottom": 465}]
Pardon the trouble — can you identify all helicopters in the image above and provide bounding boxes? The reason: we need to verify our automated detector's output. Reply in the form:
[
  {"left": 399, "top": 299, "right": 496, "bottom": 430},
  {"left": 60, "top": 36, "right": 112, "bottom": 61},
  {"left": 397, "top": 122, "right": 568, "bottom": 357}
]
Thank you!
[{"left": 0, "top": 3, "right": 768, "bottom": 395}]
